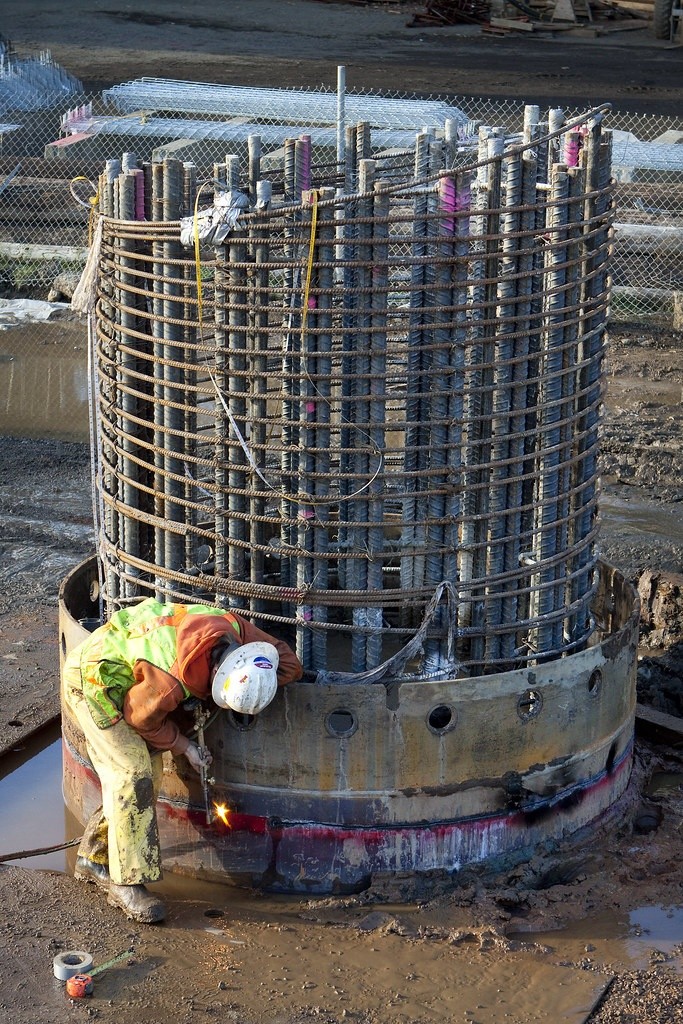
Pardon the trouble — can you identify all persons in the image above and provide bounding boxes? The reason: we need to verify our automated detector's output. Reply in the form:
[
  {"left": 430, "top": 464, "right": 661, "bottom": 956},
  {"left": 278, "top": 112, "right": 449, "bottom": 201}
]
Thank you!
[{"left": 62, "top": 597, "right": 303, "bottom": 923}]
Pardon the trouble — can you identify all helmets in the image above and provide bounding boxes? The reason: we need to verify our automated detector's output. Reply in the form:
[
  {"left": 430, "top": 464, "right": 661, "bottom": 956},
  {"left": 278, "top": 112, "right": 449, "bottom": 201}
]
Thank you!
[{"left": 212, "top": 642, "right": 280, "bottom": 715}]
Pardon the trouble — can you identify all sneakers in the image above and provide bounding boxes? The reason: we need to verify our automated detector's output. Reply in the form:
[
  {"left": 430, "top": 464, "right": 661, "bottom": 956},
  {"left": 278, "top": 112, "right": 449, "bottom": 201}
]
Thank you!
[
  {"left": 107, "top": 878, "right": 166, "bottom": 922},
  {"left": 74, "top": 854, "right": 111, "bottom": 892}
]
